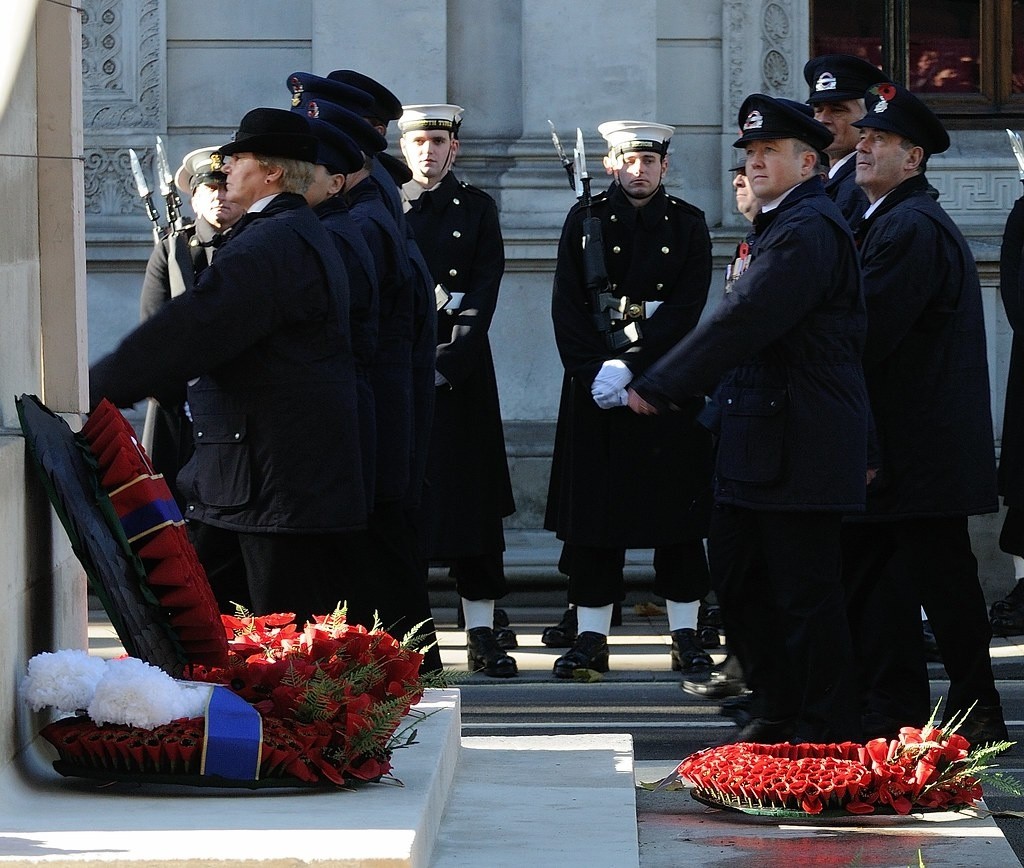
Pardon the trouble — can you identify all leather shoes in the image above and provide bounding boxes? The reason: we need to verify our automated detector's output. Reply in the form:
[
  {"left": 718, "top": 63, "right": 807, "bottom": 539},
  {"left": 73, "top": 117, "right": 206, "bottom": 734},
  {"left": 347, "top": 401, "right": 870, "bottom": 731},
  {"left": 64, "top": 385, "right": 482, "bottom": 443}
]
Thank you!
[
  {"left": 397, "top": 605, "right": 1008, "bottom": 754},
  {"left": 988, "top": 577, "right": 1024, "bottom": 636}
]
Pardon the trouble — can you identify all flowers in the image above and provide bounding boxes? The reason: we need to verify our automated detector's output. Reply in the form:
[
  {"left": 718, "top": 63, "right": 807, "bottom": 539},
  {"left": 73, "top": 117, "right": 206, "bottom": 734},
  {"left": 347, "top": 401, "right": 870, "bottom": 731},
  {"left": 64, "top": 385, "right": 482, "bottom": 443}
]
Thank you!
[
  {"left": 18, "top": 399, "right": 488, "bottom": 796},
  {"left": 677, "top": 697, "right": 1023, "bottom": 826}
]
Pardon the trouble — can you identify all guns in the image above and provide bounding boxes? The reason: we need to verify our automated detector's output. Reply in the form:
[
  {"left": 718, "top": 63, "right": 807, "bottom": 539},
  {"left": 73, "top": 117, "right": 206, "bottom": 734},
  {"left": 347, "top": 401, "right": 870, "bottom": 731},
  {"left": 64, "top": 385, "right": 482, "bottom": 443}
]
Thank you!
[
  {"left": 575, "top": 126, "right": 643, "bottom": 357},
  {"left": 1006, "top": 129, "right": 1024, "bottom": 171},
  {"left": 574, "top": 148, "right": 584, "bottom": 206},
  {"left": 128, "top": 148, "right": 166, "bottom": 244},
  {"left": 156, "top": 135, "right": 193, "bottom": 228},
  {"left": 1015, "top": 133, "right": 1024, "bottom": 186},
  {"left": 548, "top": 119, "right": 575, "bottom": 191},
  {"left": 155, "top": 144, "right": 196, "bottom": 300}
]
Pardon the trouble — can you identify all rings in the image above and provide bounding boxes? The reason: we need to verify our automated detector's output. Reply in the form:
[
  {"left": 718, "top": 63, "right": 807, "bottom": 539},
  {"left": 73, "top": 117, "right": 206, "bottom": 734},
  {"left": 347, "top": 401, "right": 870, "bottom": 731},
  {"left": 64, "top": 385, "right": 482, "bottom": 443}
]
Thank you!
[{"left": 641, "top": 405, "right": 649, "bottom": 410}]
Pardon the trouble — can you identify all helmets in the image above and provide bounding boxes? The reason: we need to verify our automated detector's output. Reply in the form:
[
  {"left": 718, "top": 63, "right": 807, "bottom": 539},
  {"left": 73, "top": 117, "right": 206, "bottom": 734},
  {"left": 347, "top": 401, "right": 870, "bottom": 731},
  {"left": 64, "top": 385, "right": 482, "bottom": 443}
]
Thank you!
[{"left": 308, "top": 119, "right": 366, "bottom": 175}]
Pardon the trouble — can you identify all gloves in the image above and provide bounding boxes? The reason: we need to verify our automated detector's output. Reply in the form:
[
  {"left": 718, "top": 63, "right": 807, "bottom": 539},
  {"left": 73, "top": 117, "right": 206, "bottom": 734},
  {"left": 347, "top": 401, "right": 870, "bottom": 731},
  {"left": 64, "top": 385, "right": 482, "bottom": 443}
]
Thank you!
[
  {"left": 593, "top": 388, "right": 629, "bottom": 409},
  {"left": 435, "top": 370, "right": 448, "bottom": 387},
  {"left": 591, "top": 359, "right": 633, "bottom": 399}
]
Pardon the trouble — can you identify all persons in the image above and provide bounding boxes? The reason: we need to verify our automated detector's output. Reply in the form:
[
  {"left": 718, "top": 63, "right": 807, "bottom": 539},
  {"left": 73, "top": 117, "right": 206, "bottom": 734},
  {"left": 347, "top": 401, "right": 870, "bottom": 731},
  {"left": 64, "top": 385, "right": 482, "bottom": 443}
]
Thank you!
[
  {"left": 88, "top": 71, "right": 518, "bottom": 676},
  {"left": 542, "top": 52, "right": 1024, "bottom": 755}
]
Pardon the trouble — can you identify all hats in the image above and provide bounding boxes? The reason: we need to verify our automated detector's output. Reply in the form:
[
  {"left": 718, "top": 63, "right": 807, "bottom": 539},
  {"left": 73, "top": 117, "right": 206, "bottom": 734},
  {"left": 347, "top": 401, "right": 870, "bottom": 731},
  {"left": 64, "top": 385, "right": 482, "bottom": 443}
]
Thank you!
[
  {"left": 286, "top": 73, "right": 374, "bottom": 123},
  {"left": 374, "top": 151, "right": 414, "bottom": 189},
  {"left": 327, "top": 70, "right": 402, "bottom": 127},
  {"left": 306, "top": 100, "right": 388, "bottom": 159},
  {"left": 183, "top": 146, "right": 232, "bottom": 178},
  {"left": 849, "top": 84, "right": 950, "bottom": 155},
  {"left": 804, "top": 54, "right": 893, "bottom": 102},
  {"left": 729, "top": 157, "right": 748, "bottom": 172},
  {"left": 398, "top": 104, "right": 464, "bottom": 135},
  {"left": 776, "top": 96, "right": 815, "bottom": 119},
  {"left": 218, "top": 108, "right": 320, "bottom": 164},
  {"left": 732, "top": 94, "right": 834, "bottom": 150},
  {"left": 174, "top": 165, "right": 203, "bottom": 196},
  {"left": 598, "top": 120, "right": 676, "bottom": 160}
]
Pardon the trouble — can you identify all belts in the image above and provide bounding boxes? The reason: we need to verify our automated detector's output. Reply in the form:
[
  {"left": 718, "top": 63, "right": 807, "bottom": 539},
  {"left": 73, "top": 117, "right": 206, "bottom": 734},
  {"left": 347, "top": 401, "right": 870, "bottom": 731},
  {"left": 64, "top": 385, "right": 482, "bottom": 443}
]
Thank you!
[
  {"left": 606, "top": 300, "right": 665, "bottom": 321},
  {"left": 441, "top": 293, "right": 466, "bottom": 310}
]
